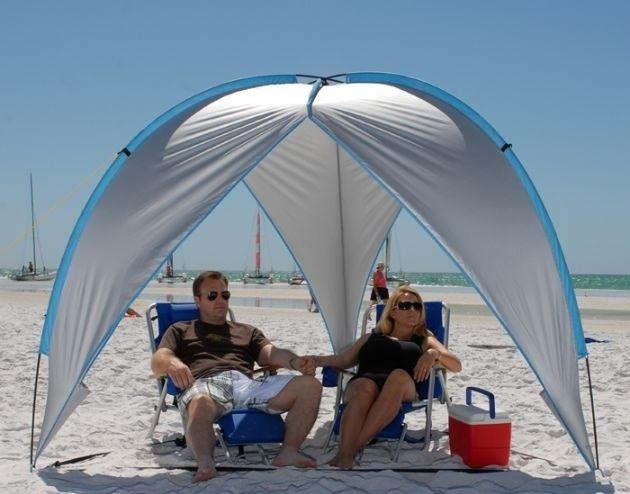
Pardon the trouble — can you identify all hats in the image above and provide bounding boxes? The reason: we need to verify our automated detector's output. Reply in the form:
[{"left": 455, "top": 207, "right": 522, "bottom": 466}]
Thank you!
[{"left": 377, "top": 262, "right": 386, "bottom": 268}]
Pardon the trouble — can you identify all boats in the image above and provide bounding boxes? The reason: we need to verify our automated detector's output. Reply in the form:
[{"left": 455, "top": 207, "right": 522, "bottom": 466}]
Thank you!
[
  {"left": 242, "top": 266, "right": 274, "bottom": 284},
  {"left": 288, "top": 277, "right": 304, "bottom": 286},
  {"left": 367, "top": 233, "right": 410, "bottom": 289},
  {"left": 156, "top": 256, "right": 188, "bottom": 283}
]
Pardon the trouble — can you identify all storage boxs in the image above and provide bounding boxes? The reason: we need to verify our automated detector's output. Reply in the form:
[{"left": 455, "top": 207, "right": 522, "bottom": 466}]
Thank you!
[{"left": 448, "top": 386, "right": 511, "bottom": 468}]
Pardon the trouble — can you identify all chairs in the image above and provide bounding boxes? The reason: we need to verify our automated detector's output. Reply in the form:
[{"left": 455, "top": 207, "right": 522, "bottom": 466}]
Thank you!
[
  {"left": 322, "top": 301, "right": 451, "bottom": 463},
  {"left": 144, "top": 302, "right": 286, "bottom": 466}
]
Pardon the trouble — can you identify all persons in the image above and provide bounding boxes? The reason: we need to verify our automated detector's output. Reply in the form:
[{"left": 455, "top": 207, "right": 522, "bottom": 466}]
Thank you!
[
  {"left": 27, "top": 261, "right": 34, "bottom": 272},
  {"left": 296, "top": 283, "right": 463, "bottom": 470},
  {"left": 367, "top": 262, "right": 390, "bottom": 322},
  {"left": 149, "top": 269, "right": 324, "bottom": 484},
  {"left": 308, "top": 296, "right": 320, "bottom": 313}
]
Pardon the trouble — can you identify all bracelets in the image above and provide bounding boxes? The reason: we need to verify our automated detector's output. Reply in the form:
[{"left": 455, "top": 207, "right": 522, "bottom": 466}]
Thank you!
[{"left": 289, "top": 357, "right": 296, "bottom": 371}]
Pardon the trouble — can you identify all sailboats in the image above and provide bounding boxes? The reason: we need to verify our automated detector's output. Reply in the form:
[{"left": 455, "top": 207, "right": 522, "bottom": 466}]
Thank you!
[{"left": 8, "top": 170, "right": 57, "bottom": 281}]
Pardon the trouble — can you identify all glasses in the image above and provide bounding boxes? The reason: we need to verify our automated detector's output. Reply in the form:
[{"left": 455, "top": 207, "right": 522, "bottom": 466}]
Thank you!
[
  {"left": 196, "top": 290, "right": 230, "bottom": 301},
  {"left": 392, "top": 299, "right": 423, "bottom": 312}
]
